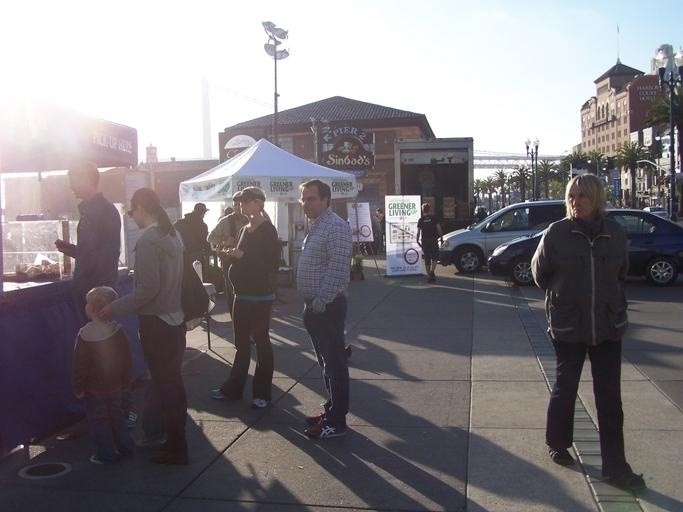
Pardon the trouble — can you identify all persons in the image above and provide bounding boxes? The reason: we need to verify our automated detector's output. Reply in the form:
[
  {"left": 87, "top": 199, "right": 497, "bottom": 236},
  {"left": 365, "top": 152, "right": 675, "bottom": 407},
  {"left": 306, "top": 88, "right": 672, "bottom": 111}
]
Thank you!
[
  {"left": 209, "top": 186, "right": 281, "bottom": 409},
  {"left": 95, "top": 185, "right": 189, "bottom": 464},
  {"left": 171, "top": 190, "right": 257, "bottom": 345},
  {"left": 374, "top": 206, "right": 385, "bottom": 233},
  {"left": 415, "top": 202, "right": 445, "bottom": 284},
  {"left": 530, "top": 173, "right": 649, "bottom": 493},
  {"left": 52, "top": 158, "right": 121, "bottom": 349},
  {"left": 72, "top": 283, "right": 135, "bottom": 468},
  {"left": 294, "top": 178, "right": 354, "bottom": 439}
]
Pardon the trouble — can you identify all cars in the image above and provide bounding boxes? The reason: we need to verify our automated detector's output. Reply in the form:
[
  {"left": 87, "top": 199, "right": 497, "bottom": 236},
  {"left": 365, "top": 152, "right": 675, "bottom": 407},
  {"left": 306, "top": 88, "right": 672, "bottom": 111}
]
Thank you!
[
  {"left": 487, "top": 208, "right": 683, "bottom": 285},
  {"left": 643, "top": 204, "right": 668, "bottom": 218}
]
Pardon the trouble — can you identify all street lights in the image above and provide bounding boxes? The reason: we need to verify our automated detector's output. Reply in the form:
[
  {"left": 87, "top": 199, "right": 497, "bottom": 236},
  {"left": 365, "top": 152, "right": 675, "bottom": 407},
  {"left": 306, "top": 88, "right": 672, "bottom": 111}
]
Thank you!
[
  {"left": 657, "top": 65, "right": 683, "bottom": 219},
  {"left": 634, "top": 159, "right": 669, "bottom": 175},
  {"left": 525, "top": 137, "right": 539, "bottom": 200},
  {"left": 262, "top": 18, "right": 290, "bottom": 151}
]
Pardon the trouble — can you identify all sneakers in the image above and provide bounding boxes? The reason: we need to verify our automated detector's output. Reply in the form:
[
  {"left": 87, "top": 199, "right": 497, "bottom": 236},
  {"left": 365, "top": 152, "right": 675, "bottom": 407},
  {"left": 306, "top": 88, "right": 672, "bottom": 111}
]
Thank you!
[
  {"left": 253, "top": 399, "right": 268, "bottom": 407},
  {"left": 306, "top": 413, "right": 346, "bottom": 439},
  {"left": 548, "top": 446, "right": 573, "bottom": 465},
  {"left": 212, "top": 389, "right": 228, "bottom": 398},
  {"left": 608, "top": 473, "right": 643, "bottom": 487}
]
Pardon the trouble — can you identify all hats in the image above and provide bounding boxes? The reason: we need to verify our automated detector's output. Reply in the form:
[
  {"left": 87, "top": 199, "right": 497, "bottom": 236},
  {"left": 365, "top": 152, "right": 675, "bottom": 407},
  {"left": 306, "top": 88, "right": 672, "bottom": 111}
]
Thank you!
[
  {"left": 195, "top": 203, "right": 209, "bottom": 212},
  {"left": 233, "top": 187, "right": 264, "bottom": 200}
]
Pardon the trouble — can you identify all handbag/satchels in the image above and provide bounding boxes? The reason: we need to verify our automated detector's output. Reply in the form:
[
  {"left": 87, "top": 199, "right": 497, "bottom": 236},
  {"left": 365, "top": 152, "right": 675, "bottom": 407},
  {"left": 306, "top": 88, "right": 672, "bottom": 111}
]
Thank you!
[{"left": 181, "top": 252, "right": 208, "bottom": 321}]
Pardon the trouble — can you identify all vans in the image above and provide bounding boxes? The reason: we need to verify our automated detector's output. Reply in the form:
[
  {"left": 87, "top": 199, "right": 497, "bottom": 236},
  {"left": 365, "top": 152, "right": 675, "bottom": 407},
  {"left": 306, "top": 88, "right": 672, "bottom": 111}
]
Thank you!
[{"left": 437, "top": 199, "right": 616, "bottom": 272}]
